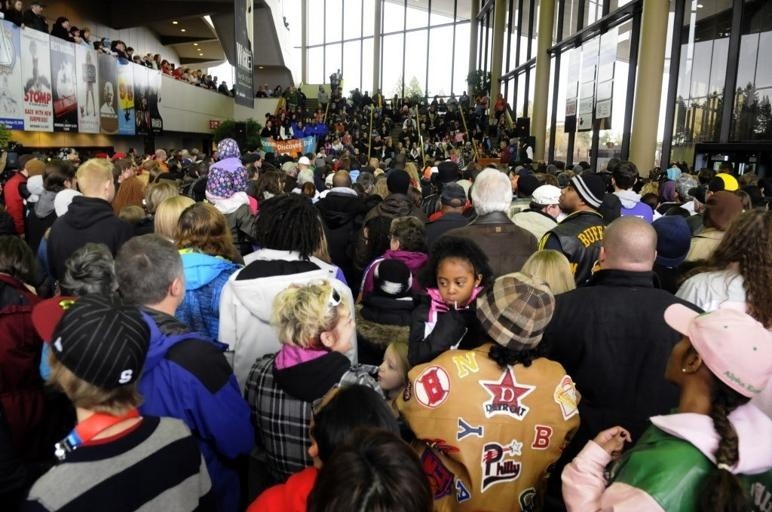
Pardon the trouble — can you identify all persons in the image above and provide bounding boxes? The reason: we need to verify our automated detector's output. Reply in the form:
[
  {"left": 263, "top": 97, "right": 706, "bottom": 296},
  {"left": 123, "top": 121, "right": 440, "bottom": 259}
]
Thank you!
[
  {"left": 0, "top": 0, "right": 237, "bottom": 96},
  {"left": 0, "top": 86, "right": 771, "bottom": 510}
]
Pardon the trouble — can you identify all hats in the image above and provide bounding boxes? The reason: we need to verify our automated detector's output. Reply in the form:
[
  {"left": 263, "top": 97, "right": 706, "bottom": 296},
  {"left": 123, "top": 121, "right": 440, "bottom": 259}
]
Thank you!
[
  {"left": 386, "top": 169, "right": 411, "bottom": 194},
  {"left": 430, "top": 162, "right": 462, "bottom": 183},
  {"left": 438, "top": 184, "right": 467, "bottom": 207},
  {"left": 570, "top": 169, "right": 604, "bottom": 211},
  {"left": 29, "top": 284, "right": 152, "bottom": 389},
  {"left": 712, "top": 172, "right": 740, "bottom": 192},
  {"left": 371, "top": 256, "right": 414, "bottom": 299},
  {"left": 654, "top": 215, "right": 692, "bottom": 266},
  {"left": 702, "top": 190, "right": 743, "bottom": 230},
  {"left": 531, "top": 184, "right": 561, "bottom": 206},
  {"left": 661, "top": 302, "right": 772, "bottom": 402},
  {"left": 473, "top": 273, "right": 557, "bottom": 350}
]
